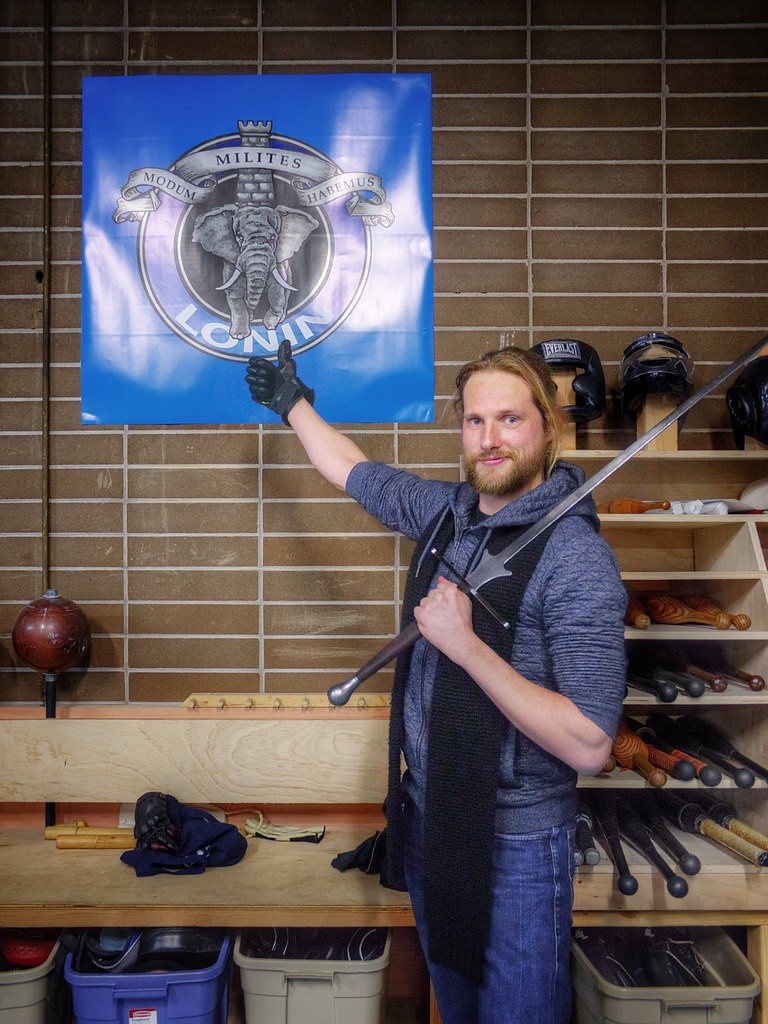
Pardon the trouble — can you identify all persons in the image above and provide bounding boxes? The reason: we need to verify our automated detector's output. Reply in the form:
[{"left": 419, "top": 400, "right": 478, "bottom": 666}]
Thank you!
[{"left": 244, "top": 339, "right": 628, "bottom": 1024}]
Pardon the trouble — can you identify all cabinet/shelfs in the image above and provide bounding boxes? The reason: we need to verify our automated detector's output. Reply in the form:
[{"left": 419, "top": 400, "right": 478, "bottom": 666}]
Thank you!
[{"left": 0, "top": 448, "right": 768, "bottom": 1024}]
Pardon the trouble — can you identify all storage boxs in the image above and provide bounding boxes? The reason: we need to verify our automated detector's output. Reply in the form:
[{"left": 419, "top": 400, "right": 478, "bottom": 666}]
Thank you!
[
  {"left": 571, "top": 927, "right": 761, "bottom": 1024},
  {"left": 232, "top": 927, "right": 394, "bottom": 1024},
  {"left": 0, "top": 935, "right": 60, "bottom": 1024},
  {"left": 61, "top": 926, "right": 232, "bottom": 1024}
]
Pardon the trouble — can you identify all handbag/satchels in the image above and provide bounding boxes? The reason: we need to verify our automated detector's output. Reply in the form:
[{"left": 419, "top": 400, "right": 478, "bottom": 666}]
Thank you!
[{"left": 118, "top": 803, "right": 263, "bottom": 838}]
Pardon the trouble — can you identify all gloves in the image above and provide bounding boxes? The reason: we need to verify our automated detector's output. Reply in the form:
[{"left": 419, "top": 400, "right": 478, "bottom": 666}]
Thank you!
[
  {"left": 245, "top": 340, "right": 315, "bottom": 427},
  {"left": 243, "top": 817, "right": 326, "bottom": 844}
]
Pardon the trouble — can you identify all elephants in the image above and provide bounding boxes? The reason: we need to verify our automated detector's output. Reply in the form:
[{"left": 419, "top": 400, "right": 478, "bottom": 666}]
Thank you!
[{"left": 192, "top": 205, "right": 319, "bottom": 339}]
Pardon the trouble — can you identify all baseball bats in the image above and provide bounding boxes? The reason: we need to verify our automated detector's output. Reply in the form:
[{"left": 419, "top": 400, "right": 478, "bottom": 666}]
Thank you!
[{"left": 574, "top": 492, "right": 768, "bottom": 899}]
